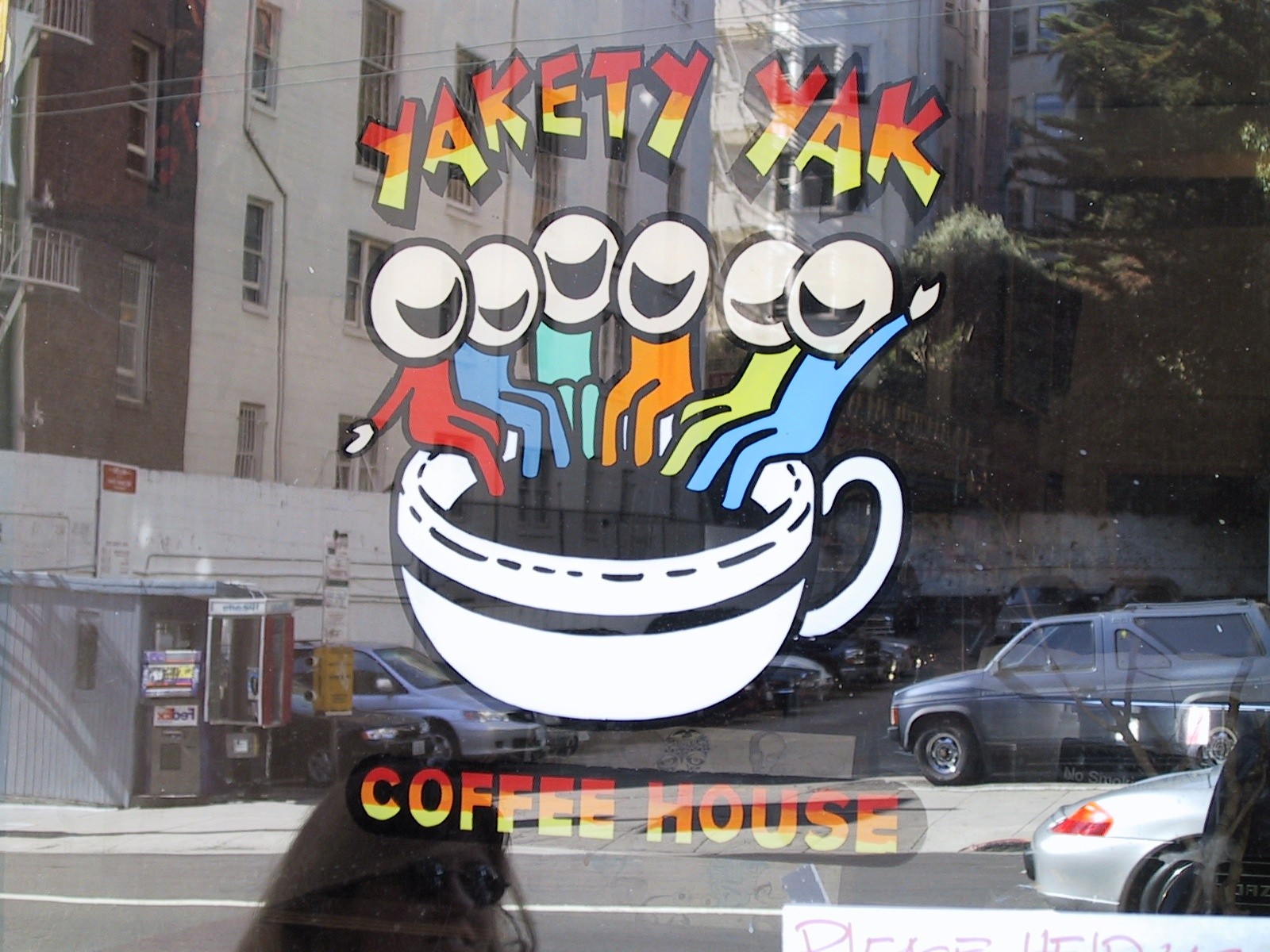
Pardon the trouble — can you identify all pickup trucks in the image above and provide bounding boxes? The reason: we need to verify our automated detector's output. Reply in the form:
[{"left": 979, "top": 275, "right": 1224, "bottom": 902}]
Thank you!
[{"left": 884, "top": 595, "right": 1270, "bottom": 787}]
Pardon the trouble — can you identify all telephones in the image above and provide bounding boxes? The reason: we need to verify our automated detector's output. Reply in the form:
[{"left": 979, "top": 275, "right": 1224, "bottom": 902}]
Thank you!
[{"left": 251, "top": 677, "right": 258, "bottom": 694}]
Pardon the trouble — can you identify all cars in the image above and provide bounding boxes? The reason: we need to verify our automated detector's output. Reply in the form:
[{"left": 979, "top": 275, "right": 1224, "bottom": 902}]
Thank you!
[
  {"left": 287, "top": 638, "right": 550, "bottom": 772},
  {"left": 992, "top": 573, "right": 1086, "bottom": 645},
  {"left": 1091, "top": 573, "right": 1183, "bottom": 613},
  {"left": 850, "top": 587, "right": 921, "bottom": 638},
  {"left": 1018, "top": 758, "right": 1270, "bottom": 917},
  {"left": 213, "top": 665, "right": 432, "bottom": 788},
  {"left": 742, "top": 636, "right": 921, "bottom": 703}
]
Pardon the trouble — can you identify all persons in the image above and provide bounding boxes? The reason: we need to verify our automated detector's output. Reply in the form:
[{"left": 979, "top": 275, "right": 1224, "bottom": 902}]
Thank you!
[{"left": 236, "top": 731, "right": 541, "bottom": 952}]
